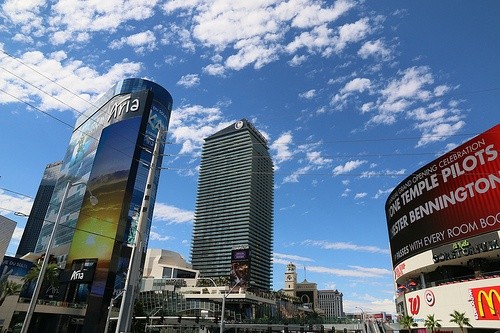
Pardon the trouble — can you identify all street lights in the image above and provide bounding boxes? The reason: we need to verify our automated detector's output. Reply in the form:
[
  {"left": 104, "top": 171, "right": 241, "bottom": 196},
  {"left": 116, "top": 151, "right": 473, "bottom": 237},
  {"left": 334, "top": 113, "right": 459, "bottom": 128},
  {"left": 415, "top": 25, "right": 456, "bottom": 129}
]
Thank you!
[
  {"left": 196, "top": 277, "right": 255, "bottom": 333},
  {"left": 21, "top": 179, "right": 99, "bottom": 333},
  {"left": 355, "top": 306, "right": 366, "bottom": 324}
]
